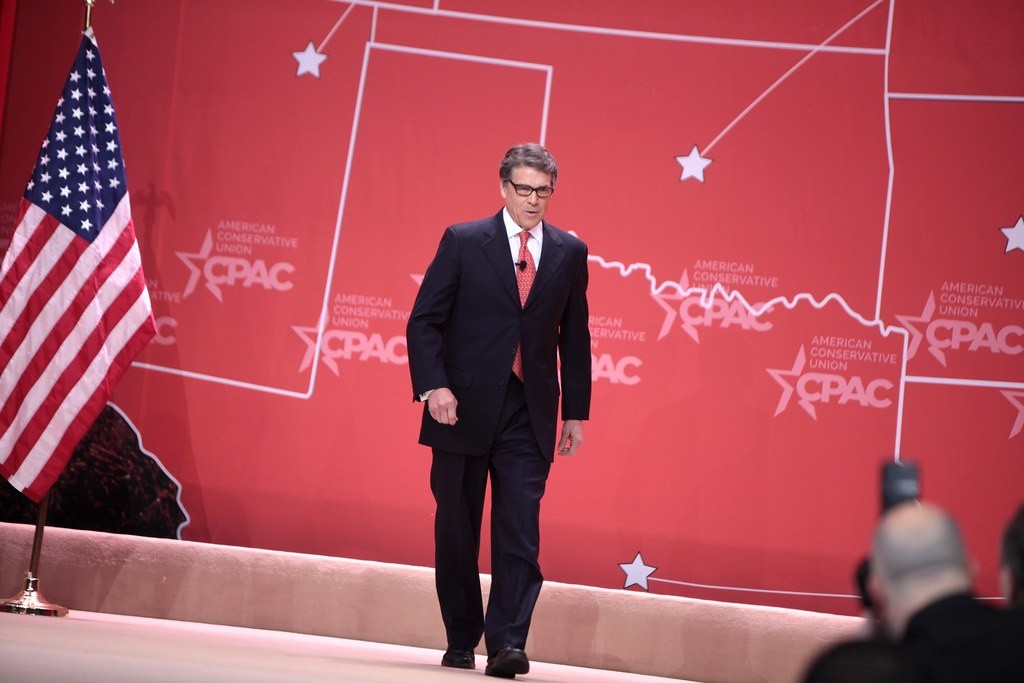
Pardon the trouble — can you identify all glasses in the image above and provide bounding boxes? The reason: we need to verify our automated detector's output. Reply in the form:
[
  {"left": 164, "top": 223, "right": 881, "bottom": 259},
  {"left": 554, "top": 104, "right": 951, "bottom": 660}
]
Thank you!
[{"left": 508, "top": 180, "right": 554, "bottom": 198}]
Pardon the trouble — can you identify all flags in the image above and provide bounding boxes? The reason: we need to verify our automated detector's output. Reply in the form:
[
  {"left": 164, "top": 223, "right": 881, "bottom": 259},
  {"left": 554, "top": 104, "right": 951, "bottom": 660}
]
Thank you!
[{"left": 0, "top": 28, "right": 158, "bottom": 502}]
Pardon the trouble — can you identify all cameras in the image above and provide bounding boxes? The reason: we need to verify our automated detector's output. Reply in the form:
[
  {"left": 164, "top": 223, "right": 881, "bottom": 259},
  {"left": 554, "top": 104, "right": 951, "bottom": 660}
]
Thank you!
[{"left": 854, "top": 462, "right": 921, "bottom": 610}]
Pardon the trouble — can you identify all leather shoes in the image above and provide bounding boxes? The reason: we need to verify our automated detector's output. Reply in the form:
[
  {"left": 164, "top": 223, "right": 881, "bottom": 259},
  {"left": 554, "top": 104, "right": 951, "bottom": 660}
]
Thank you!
[
  {"left": 442, "top": 645, "right": 475, "bottom": 669},
  {"left": 485, "top": 647, "right": 529, "bottom": 679}
]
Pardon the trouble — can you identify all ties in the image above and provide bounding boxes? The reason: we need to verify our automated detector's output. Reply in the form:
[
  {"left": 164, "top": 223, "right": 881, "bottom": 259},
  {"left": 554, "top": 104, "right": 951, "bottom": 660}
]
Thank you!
[{"left": 512, "top": 232, "right": 537, "bottom": 384}]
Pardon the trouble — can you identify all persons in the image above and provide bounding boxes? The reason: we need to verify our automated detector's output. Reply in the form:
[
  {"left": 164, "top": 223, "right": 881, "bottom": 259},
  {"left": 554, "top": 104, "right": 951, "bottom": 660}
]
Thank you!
[
  {"left": 798, "top": 500, "right": 1024, "bottom": 683},
  {"left": 406, "top": 143, "right": 592, "bottom": 679}
]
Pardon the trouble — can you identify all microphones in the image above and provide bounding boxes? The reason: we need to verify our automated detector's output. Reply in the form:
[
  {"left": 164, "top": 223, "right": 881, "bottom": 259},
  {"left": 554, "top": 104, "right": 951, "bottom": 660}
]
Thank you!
[{"left": 520, "top": 261, "right": 526, "bottom": 271}]
